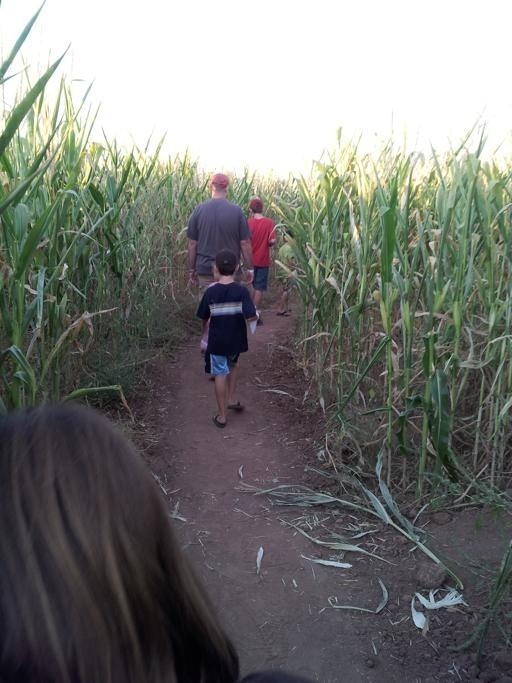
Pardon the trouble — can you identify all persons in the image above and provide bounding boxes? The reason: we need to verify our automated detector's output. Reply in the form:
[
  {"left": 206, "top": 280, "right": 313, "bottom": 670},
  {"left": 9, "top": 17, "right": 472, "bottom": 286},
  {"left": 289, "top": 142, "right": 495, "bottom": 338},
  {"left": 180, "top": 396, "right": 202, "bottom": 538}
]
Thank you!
[
  {"left": 246, "top": 199, "right": 277, "bottom": 326},
  {"left": 186, "top": 173, "right": 254, "bottom": 357},
  {"left": 0, "top": 402, "right": 311, "bottom": 683},
  {"left": 195, "top": 250, "right": 258, "bottom": 428},
  {"left": 275, "top": 230, "right": 298, "bottom": 317}
]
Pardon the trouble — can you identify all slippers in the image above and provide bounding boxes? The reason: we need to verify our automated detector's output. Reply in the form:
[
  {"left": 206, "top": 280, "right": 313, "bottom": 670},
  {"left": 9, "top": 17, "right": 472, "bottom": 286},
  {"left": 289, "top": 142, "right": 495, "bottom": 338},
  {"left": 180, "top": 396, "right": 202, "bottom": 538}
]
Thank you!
[
  {"left": 228, "top": 400, "right": 244, "bottom": 410},
  {"left": 276, "top": 310, "right": 292, "bottom": 316},
  {"left": 212, "top": 414, "right": 226, "bottom": 428}
]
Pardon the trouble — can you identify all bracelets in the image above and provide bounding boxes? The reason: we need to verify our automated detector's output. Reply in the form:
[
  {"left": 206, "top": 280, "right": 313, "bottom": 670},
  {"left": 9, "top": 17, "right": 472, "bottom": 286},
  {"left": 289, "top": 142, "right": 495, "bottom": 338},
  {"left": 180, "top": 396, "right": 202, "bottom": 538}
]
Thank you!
[{"left": 247, "top": 269, "right": 254, "bottom": 273}]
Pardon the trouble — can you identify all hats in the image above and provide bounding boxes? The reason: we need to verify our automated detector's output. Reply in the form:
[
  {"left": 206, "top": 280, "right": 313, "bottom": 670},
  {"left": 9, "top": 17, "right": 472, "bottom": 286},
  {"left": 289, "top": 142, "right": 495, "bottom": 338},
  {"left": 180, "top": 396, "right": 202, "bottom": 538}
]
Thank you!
[
  {"left": 212, "top": 175, "right": 228, "bottom": 186},
  {"left": 251, "top": 199, "right": 263, "bottom": 209}
]
